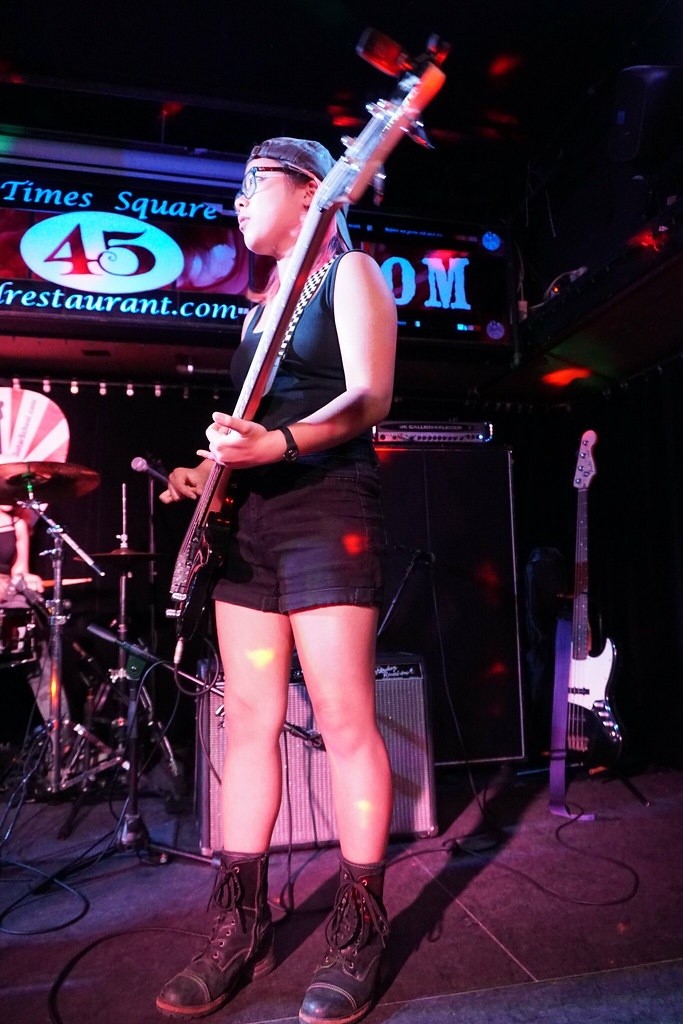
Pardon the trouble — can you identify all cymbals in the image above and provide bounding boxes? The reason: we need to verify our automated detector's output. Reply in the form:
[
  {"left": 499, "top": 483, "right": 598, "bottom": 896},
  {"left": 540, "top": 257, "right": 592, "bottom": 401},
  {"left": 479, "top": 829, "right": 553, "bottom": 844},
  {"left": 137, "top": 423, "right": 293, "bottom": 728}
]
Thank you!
[
  {"left": 0, "top": 460, "right": 102, "bottom": 503},
  {"left": 73, "top": 548, "right": 163, "bottom": 565}
]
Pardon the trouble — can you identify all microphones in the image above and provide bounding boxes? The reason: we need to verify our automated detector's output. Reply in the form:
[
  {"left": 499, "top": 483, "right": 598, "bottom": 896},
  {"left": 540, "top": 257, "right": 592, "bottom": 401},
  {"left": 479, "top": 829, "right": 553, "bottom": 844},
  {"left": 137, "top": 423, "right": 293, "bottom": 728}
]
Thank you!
[
  {"left": 302, "top": 729, "right": 324, "bottom": 749},
  {"left": 130, "top": 457, "right": 169, "bottom": 484}
]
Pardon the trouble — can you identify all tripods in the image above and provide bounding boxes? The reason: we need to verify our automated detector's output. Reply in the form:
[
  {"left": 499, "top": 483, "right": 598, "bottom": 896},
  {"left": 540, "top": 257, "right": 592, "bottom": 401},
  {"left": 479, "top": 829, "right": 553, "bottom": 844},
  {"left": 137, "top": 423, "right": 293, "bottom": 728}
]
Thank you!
[{"left": 0, "top": 475, "right": 319, "bottom": 894}]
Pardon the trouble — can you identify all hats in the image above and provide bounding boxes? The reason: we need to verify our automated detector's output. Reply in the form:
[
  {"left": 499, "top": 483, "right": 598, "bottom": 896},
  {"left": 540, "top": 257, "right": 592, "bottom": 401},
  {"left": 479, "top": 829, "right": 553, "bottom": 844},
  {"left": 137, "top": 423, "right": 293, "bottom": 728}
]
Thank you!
[{"left": 251, "top": 137, "right": 356, "bottom": 252}]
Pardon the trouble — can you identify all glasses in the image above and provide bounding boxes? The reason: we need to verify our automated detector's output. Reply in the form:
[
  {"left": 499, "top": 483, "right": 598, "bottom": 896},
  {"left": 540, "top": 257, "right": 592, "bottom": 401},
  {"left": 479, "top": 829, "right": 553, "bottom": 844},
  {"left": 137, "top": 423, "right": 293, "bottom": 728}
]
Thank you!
[{"left": 234, "top": 165, "right": 312, "bottom": 216}]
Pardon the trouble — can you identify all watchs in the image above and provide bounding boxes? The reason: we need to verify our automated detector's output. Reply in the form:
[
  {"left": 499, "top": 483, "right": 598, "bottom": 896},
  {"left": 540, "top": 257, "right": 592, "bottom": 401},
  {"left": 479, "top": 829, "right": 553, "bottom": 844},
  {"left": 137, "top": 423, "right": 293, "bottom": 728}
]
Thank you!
[{"left": 279, "top": 425, "right": 299, "bottom": 462}]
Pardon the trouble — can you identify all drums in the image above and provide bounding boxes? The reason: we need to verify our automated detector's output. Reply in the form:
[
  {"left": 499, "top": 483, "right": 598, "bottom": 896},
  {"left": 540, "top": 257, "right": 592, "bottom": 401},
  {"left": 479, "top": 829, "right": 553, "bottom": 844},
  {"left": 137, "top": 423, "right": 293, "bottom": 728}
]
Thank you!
[{"left": 0, "top": 571, "right": 42, "bottom": 670}]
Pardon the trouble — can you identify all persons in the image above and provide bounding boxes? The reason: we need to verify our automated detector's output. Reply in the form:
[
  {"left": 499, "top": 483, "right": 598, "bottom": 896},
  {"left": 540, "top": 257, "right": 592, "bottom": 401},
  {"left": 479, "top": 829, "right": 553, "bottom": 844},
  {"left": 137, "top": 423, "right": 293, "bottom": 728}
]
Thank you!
[
  {"left": 159, "top": 139, "right": 395, "bottom": 1024},
  {"left": 0, "top": 500, "right": 83, "bottom": 782}
]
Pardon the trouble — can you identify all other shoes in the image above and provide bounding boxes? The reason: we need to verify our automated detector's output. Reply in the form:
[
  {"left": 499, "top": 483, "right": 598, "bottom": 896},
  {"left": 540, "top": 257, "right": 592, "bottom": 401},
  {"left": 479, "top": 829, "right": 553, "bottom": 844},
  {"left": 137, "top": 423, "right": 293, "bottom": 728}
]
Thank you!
[{"left": 46, "top": 746, "right": 109, "bottom": 785}]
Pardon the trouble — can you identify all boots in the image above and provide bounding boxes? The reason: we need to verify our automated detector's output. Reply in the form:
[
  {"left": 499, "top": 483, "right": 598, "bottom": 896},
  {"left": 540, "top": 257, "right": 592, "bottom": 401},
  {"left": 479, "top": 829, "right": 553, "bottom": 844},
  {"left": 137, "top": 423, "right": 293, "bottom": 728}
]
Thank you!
[
  {"left": 295, "top": 852, "right": 392, "bottom": 1024},
  {"left": 154, "top": 845, "right": 275, "bottom": 1020}
]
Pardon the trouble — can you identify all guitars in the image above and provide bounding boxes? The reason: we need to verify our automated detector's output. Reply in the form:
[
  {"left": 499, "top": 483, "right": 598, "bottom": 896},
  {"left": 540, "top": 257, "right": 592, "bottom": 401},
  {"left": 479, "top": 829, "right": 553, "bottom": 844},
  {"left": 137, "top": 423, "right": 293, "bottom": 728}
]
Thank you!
[
  {"left": 545, "top": 429, "right": 623, "bottom": 781},
  {"left": 165, "top": 28, "right": 449, "bottom": 654}
]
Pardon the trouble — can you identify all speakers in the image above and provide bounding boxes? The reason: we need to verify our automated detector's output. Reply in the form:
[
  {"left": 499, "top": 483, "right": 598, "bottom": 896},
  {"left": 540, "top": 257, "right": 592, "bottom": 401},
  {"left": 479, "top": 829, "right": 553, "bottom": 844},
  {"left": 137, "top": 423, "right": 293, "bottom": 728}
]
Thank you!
[
  {"left": 365, "top": 443, "right": 533, "bottom": 773},
  {"left": 610, "top": 65, "right": 683, "bottom": 173},
  {"left": 194, "top": 652, "right": 440, "bottom": 855}
]
